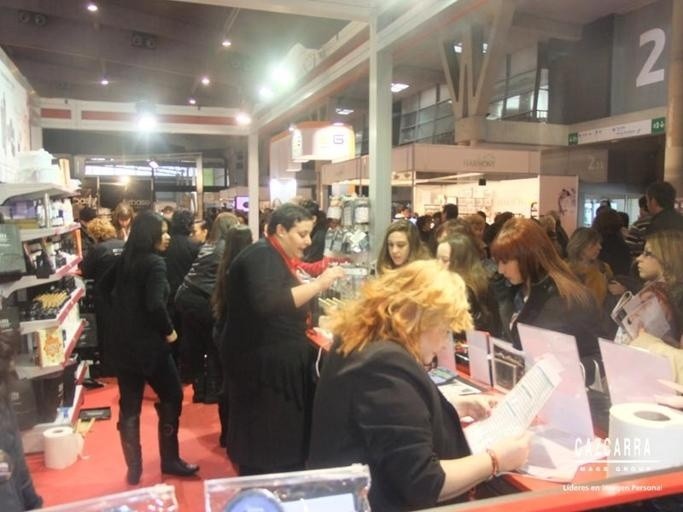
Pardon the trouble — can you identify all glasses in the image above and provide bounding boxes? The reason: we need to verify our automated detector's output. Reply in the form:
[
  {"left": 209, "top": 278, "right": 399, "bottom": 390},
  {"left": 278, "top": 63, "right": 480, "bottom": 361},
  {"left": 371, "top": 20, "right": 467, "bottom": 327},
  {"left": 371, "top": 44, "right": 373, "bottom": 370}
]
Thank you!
[{"left": 640, "top": 248, "right": 663, "bottom": 266}]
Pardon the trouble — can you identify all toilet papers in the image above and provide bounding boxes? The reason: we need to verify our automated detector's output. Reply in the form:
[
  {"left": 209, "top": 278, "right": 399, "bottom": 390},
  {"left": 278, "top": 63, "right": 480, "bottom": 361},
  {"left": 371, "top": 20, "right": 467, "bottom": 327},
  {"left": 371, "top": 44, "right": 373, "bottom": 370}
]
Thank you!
[{"left": 607, "top": 401, "right": 683, "bottom": 482}]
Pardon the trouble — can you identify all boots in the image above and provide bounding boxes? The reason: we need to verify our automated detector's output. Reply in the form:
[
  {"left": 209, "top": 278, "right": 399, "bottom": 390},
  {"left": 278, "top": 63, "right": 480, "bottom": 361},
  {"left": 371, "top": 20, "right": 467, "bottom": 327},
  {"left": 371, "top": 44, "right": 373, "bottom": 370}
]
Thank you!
[
  {"left": 193, "top": 374, "right": 222, "bottom": 403},
  {"left": 155, "top": 401, "right": 199, "bottom": 475},
  {"left": 118, "top": 398, "right": 143, "bottom": 484}
]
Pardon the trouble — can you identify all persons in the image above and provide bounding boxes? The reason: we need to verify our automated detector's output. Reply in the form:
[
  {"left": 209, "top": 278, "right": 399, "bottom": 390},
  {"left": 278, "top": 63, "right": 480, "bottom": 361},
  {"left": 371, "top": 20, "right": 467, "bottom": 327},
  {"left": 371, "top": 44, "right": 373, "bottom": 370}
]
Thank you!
[{"left": 79, "top": 181, "right": 682, "bottom": 509}]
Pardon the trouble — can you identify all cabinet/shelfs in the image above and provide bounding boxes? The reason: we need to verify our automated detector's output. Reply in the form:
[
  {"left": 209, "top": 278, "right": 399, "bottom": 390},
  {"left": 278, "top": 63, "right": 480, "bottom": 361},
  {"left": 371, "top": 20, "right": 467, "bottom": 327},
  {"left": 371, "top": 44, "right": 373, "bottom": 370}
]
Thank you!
[{"left": 0, "top": 180, "right": 88, "bottom": 454}]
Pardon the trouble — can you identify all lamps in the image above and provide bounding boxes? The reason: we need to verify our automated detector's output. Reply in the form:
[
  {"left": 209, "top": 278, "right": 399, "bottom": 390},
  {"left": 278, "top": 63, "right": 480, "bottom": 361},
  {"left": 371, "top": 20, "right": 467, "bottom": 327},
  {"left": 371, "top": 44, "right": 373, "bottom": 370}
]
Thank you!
[{"left": 290, "top": 103, "right": 355, "bottom": 163}]
